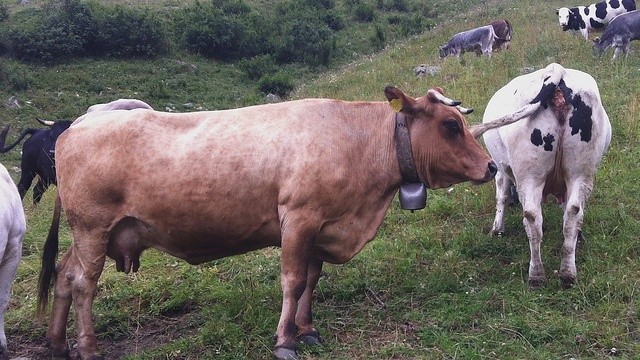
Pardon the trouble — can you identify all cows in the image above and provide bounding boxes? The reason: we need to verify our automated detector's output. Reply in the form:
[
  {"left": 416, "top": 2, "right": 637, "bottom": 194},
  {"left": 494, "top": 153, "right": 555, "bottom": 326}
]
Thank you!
[
  {"left": 0, "top": 164, "right": 25, "bottom": 356},
  {"left": 461, "top": 18, "right": 512, "bottom": 57},
  {"left": 438, "top": 24, "right": 505, "bottom": 61},
  {"left": 0, "top": 116, "right": 75, "bottom": 206},
  {"left": 34, "top": 84, "right": 498, "bottom": 360},
  {"left": 554, "top": 0, "right": 637, "bottom": 41},
  {"left": 590, "top": 9, "right": 640, "bottom": 62},
  {"left": 465, "top": 62, "right": 613, "bottom": 290}
]
